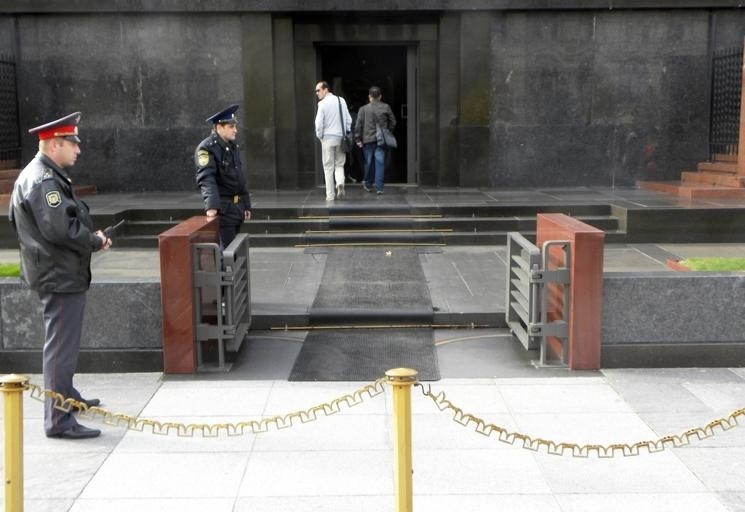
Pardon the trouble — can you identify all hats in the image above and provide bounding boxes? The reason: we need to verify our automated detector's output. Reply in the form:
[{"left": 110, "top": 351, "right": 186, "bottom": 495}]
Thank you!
[
  {"left": 27, "top": 111, "right": 83, "bottom": 143},
  {"left": 205, "top": 104, "right": 241, "bottom": 125}
]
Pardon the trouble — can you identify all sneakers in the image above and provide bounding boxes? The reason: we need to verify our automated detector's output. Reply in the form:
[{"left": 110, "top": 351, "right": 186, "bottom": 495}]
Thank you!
[{"left": 362, "top": 181, "right": 384, "bottom": 195}]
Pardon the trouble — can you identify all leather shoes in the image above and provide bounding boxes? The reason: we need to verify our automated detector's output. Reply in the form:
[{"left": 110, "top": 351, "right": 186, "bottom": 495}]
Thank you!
[
  {"left": 336, "top": 183, "right": 346, "bottom": 201},
  {"left": 46, "top": 398, "right": 101, "bottom": 439}
]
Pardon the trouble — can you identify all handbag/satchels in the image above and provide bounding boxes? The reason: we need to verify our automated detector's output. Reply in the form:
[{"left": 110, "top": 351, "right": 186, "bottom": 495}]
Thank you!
[
  {"left": 341, "top": 132, "right": 354, "bottom": 153},
  {"left": 375, "top": 123, "right": 397, "bottom": 150}
]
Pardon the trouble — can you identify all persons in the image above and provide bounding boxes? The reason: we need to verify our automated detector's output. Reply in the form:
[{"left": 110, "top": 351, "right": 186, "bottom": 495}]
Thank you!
[
  {"left": 355, "top": 85, "right": 397, "bottom": 195},
  {"left": 7, "top": 109, "right": 114, "bottom": 440},
  {"left": 194, "top": 102, "right": 253, "bottom": 300},
  {"left": 314, "top": 81, "right": 353, "bottom": 201}
]
